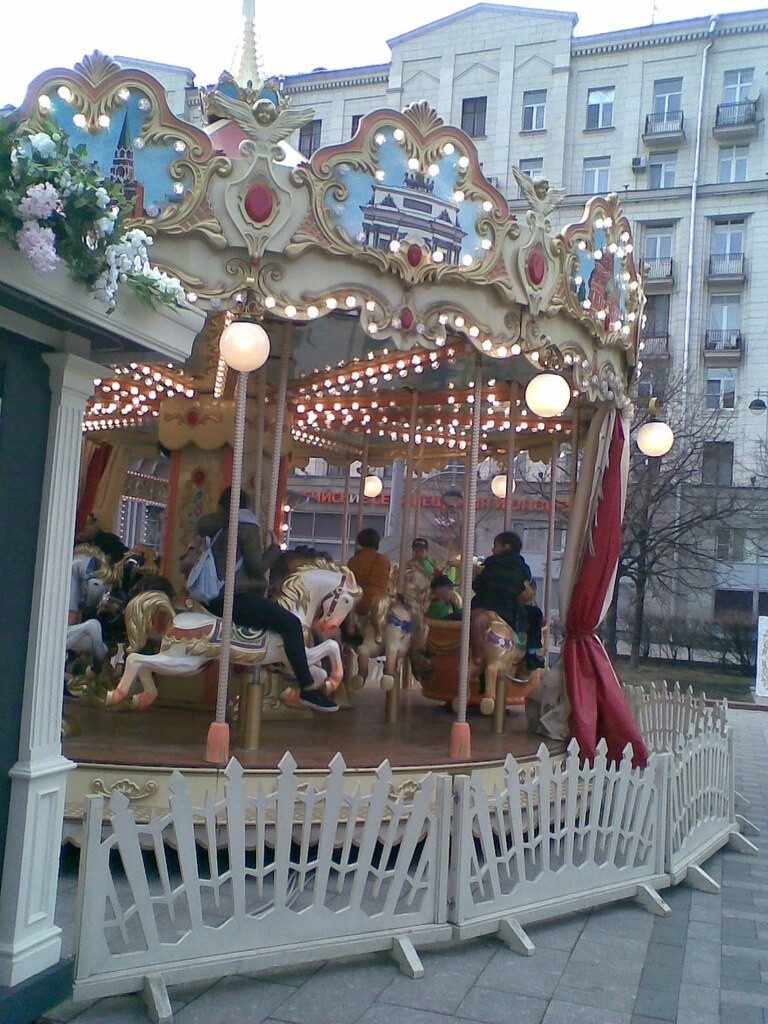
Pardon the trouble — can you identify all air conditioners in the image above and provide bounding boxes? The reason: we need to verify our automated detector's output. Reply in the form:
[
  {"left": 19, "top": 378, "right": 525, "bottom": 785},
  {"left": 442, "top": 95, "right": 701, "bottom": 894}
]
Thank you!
[
  {"left": 631, "top": 155, "right": 647, "bottom": 168},
  {"left": 486, "top": 176, "right": 498, "bottom": 187}
]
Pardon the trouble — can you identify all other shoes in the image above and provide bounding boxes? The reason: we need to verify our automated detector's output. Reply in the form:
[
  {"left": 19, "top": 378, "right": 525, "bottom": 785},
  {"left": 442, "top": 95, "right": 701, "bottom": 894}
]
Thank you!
[
  {"left": 297, "top": 688, "right": 339, "bottom": 712},
  {"left": 527, "top": 652, "right": 546, "bottom": 669}
]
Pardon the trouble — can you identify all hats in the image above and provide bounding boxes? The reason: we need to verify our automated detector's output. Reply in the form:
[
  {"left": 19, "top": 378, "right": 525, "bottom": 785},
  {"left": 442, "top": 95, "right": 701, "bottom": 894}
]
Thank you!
[
  {"left": 429, "top": 574, "right": 460, "bottom": 588},
  {"left": 412, "top": 539, "right": 428, "bottom": 551},
  {"left": 92, "top": 509, "right": 113, "bottom": 534},
  {"left": 356, "top": 528, "right": 380, "bottom": 550}
]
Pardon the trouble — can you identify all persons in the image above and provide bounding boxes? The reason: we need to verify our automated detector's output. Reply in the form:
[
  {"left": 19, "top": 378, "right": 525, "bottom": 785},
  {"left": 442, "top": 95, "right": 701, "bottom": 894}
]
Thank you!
[
  {"left": 73, "top": 512, "right": 133, "bottom": 567},
  {"left": 468, "top": 531, "right": 546, "bottom": 670},
  {"left": 195, "top": 485, "right": 340, "bottom": 711},
  {"left": 410, "top": 538, "right": 443, "bottom": 582},
  {"left": 343, "top": 528, "right": 391, "bottom": 649},
  {"left": 427, "top": 575, "right": 465, "bottom": 621}
]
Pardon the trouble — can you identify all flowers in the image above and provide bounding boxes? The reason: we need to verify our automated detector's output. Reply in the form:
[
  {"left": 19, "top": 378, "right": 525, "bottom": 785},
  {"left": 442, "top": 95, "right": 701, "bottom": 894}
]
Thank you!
[{"left": 0, "top": 105, "right": 192, "bottom": 316}]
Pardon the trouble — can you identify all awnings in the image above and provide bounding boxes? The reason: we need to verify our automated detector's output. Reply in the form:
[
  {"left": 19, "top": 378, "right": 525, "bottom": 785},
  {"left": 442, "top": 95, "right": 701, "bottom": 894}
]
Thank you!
[{"left": 515, "top": 544, "right": 768, "bottom": 594}]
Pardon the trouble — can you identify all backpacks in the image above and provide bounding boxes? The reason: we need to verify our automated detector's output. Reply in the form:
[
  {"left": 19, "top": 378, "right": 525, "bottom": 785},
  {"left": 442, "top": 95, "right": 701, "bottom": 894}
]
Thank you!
[{"left": 185, "top": 528, "right": 244, "bottom": 603}]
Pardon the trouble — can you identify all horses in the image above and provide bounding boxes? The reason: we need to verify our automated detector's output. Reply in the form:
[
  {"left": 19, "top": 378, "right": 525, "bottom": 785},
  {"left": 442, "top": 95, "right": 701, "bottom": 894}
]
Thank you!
[{"left": 69, "top": 540, "right": 548, "bottom": 716}]
